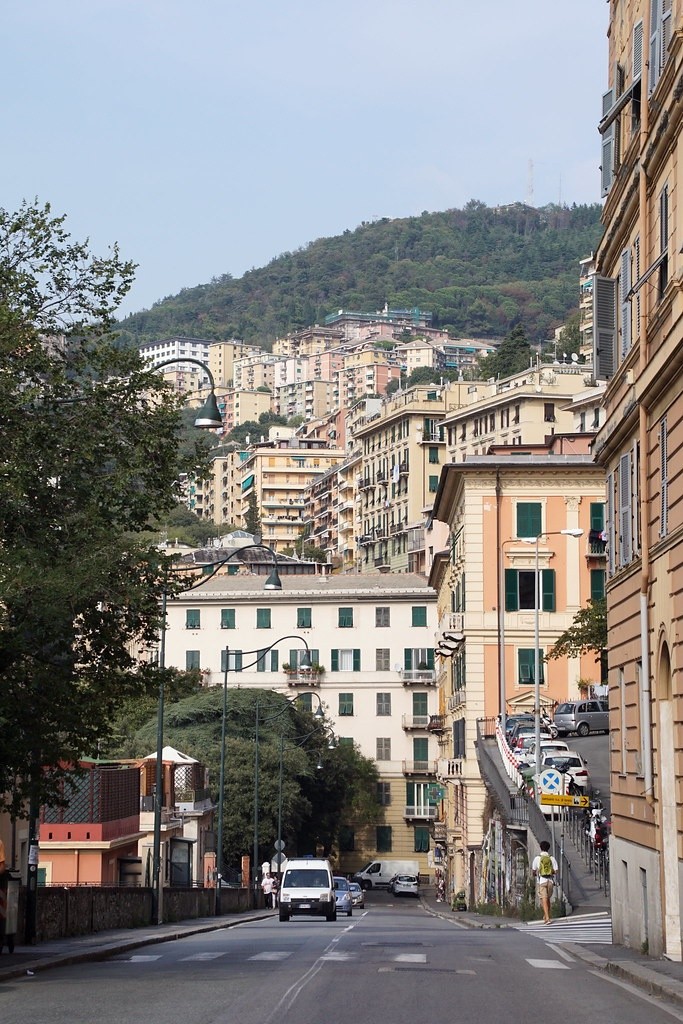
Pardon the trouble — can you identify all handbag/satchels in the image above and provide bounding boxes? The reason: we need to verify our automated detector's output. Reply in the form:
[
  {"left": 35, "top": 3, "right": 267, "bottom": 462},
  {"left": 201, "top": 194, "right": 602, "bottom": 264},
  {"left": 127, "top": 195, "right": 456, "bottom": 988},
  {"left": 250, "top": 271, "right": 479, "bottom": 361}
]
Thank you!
[{"left": 538, "top": 855, "right": 553, "bottom": 877}]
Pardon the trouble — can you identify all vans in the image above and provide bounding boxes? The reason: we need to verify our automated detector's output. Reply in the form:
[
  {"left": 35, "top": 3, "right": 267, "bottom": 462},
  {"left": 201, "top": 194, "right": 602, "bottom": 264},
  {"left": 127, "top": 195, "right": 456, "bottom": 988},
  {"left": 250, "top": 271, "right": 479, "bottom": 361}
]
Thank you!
[
  {"left": 273, "top": 857, "right": 338, "bottom": 922},
  {"left": 353, "top": 860, "right": 420, "bottom": 891}
]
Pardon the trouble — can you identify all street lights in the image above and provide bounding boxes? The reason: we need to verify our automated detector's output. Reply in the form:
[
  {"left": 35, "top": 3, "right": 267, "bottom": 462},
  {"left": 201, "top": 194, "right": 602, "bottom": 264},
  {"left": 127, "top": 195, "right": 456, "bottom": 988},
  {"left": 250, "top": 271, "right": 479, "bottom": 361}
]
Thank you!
[
  {"left": 254, "top": 692, "right": 325, "bottom": 911},
  {"left": 534, "top": 528, "right": 587, "bottom": 806},
  {"left": 150, "top": 544, "right": 285, "bottom": 927},
  {"left": 277, "top": 726, "right": 337, "bottom": 886},
  {"left": 25, "top": 358, "right": 225, "bottom": 949},
  {"left": 213, "top": 636, "right": 313, "bottom": 918}
]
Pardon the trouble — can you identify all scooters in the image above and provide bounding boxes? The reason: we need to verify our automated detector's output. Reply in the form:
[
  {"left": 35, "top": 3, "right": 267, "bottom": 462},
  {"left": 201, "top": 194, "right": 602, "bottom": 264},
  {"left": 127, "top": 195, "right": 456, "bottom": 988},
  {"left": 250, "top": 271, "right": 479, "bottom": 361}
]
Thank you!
[
  {"left": 587, "top": 789, "right": 612, "bottom": 866},
  {"left": 551, "top": 761, "right": 583, "bottom": 796}
]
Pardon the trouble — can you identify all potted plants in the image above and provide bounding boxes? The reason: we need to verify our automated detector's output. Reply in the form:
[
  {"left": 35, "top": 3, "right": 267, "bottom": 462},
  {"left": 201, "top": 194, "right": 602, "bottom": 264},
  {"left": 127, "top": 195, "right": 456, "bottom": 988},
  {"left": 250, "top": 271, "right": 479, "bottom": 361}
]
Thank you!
[
  {"left": 283, "top": 663, "right": 324, "bottom": 674},
  {"left": 417, "top": 662, "right": 428, "bottom": 679}
]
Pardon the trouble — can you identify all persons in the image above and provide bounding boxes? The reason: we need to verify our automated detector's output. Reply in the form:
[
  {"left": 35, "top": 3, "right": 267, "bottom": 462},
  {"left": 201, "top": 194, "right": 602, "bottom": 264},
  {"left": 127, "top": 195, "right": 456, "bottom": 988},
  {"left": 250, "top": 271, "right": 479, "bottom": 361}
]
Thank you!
[
  {"left": 435, "top": 873, "right": 445, "bottom": 902},
  {"left": 532, "top": 841, "right": 558, "bottom": 925},
  {"left": 261, "top": 873, "right": 281, "bottom": 910},
  {"left": 0, "top": 840, "right": 14, "bottom": 953}
]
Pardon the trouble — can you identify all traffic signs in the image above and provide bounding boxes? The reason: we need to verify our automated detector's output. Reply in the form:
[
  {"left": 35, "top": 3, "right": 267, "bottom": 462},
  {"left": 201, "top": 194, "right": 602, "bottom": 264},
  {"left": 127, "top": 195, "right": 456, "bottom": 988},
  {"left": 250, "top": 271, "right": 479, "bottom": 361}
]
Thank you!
[{"left": 541, "top": 794, "right": 590, "bottom": 807}]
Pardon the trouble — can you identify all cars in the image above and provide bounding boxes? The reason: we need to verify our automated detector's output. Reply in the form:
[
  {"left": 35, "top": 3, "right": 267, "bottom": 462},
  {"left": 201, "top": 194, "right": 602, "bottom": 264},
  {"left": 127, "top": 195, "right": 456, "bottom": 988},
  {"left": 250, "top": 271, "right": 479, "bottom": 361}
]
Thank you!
[
  {"left": 389, "top": 872, "right": 420, "bottom": 898},
  {"left": 332, "top": 876, "right": 355, "bottom": 916},
  {"left": 505, "top": 714, "right": 570, "bottom": 766},
  {"left": 349, "top": 883, "right": 366, "bottom": 909}
]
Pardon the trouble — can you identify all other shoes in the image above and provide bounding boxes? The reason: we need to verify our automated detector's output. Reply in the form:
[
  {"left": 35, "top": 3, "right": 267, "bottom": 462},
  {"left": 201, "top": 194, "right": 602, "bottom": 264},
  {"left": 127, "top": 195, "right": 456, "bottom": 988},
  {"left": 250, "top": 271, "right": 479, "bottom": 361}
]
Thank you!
[
  {"left": 543, "top": 919, "right": 551, "bottom": 925},
  {"left": 543, "top": 916, "right": 546, "bottom": 920}
]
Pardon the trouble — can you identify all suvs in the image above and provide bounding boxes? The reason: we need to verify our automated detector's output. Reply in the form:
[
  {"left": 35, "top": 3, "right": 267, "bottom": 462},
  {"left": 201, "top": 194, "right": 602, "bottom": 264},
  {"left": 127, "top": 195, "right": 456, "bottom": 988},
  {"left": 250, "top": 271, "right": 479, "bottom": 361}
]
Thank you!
[
  {"left": 541, "top": 750, "right": 592, "bottom": 796},
  {"left": 553, "top": 700, "right": 610, "bottom": 737}
]
